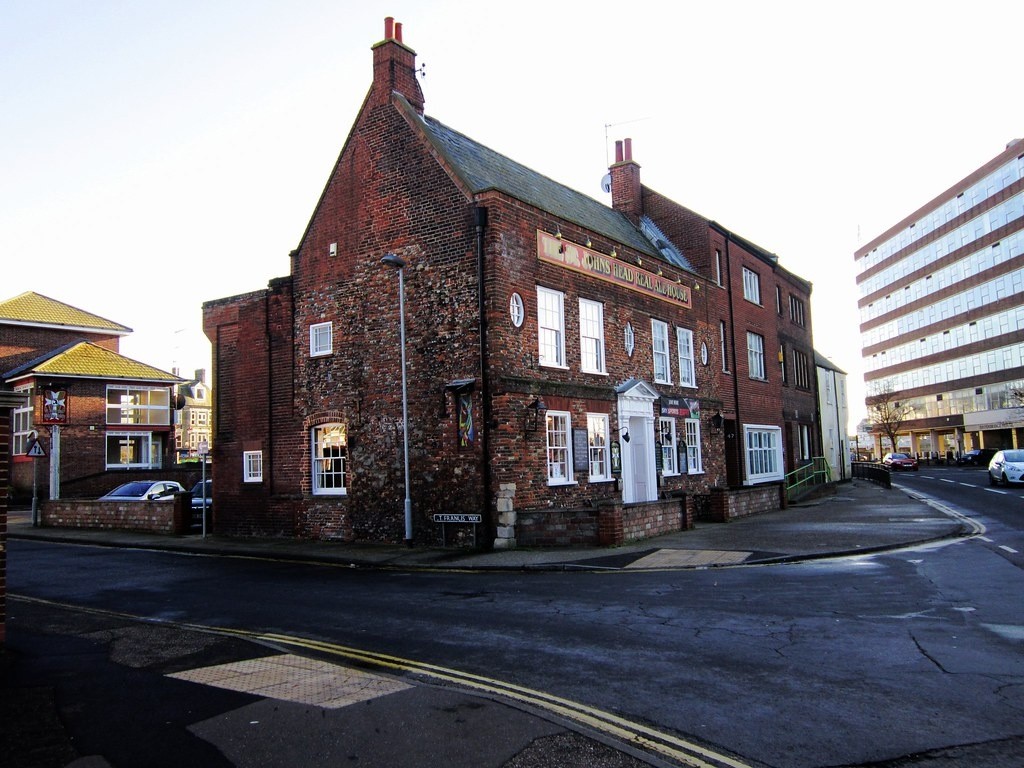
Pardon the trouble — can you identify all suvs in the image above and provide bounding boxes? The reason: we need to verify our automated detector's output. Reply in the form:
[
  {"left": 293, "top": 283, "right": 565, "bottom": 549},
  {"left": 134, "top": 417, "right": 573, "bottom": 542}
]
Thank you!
[{"left": 187, "top": 478, "right": 213, "bottom": 533}]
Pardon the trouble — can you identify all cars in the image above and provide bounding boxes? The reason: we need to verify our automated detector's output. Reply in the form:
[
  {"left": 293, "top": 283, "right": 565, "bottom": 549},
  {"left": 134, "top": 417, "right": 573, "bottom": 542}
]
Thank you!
[
  {"left": 99, "top": 480, "right": 187, "bottom": 501},
  {"left": 961, "top": 449, "right": 998, "bottom": 467},
  {"left": 988, "top": 450, "right": 1024, "bottom": 490},
  {"left": 883, "top": 452, "right": 918, "bottom": 472}
]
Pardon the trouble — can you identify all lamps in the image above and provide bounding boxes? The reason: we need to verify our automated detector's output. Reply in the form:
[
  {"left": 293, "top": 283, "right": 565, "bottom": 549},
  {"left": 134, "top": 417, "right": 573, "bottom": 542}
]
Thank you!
[
  {"left": 655, "top": 426, "right": 673, "bottom": 441},
  {"left": 521, "top": 397, "right": 547, "bottom": 436},
  {"left": 613, "top": 426, "right": 630, "bottom": 442},
  {"left": 708, "top": 411, "right": 722, "bottom": 436}
]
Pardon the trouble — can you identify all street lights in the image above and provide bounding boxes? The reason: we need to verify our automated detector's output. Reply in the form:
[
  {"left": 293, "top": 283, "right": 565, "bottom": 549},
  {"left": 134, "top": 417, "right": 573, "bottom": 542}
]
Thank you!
[{"left": 379, "top": 256, "right": 414, "bottom": 549}]
